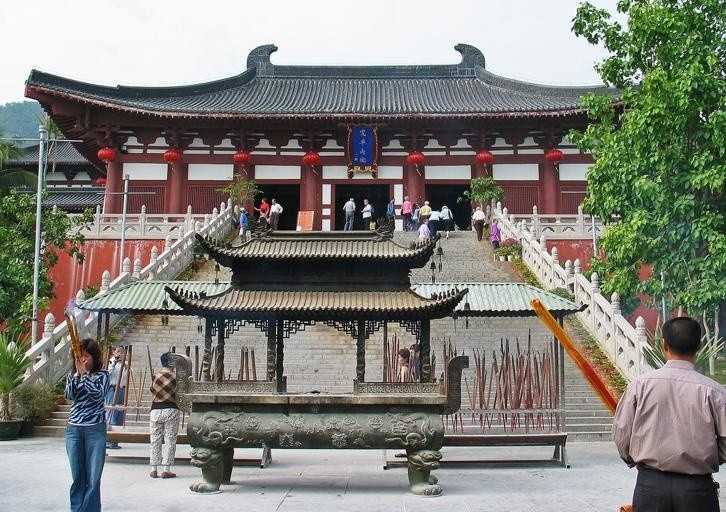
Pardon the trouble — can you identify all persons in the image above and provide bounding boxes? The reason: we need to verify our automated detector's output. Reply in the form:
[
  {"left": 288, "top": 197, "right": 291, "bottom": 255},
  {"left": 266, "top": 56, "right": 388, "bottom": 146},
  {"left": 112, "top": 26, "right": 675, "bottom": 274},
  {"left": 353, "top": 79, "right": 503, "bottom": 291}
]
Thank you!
[
  {"left": 104, "top": 344, "right": 134, "bottom": 449},
  {"left": 148, "top": 352, "right": 181, "bottom": 479},
  {"left": 610, "top": 316, "right": 726, "bottom": 512},
  {"left": 63, "top": 338, "right": 111, "bottom": 512},
  {"left": 410, "top": 342, "right": 421, "bottom": 382},
  {"left": 257, "top": 208, "right": 269, "bottom": 222},
  {"left": 254, "top": 197, "right": 270, "bottom": 223},
  {"left": 238, "top": 207, "right": 249, "bottom": 243},
  {"left": 341, "top": 195, "right": 501, "bottom": 250},
  {"left": 269, "top": 198, "right": 284, "bottom": 230},
  {"left": 394, "top": 347, "right": 411, "bottom": 457}
]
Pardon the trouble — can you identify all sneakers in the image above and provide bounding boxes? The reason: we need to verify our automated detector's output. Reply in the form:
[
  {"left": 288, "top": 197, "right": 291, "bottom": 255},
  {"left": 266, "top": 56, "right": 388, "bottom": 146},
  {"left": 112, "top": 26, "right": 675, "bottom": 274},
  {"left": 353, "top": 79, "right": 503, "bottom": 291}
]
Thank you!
[
  {"left": 162, "top": 472, "right": 176, "bottom": 478},
  {"left": 150, "top": 471, "right": 157, "bottom": 477}
]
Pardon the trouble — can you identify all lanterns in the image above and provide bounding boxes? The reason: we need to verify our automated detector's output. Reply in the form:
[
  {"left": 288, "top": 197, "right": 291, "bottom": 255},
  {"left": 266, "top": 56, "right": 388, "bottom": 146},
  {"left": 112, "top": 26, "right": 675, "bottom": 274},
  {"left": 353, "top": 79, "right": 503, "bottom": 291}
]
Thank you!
[
  {"left": 231, "top": 151, "right": 254, "bottom": 174},
  {"left": 95, "top": 177, "right": 106, "bottom": 186},
  {"left": 407, "top": 151, "right": 425, "bottom": 176},
  {"left": 163, "top": 148, "right": 183, "bottom": 172},
  {"left": 545, "top": 149, "right": 564, "bottom": 173},
  {"left": 300, "top": 150, "right": 321, "bottom": 176},
  {"left": 97, "top": 147, "right": 116, "bottom": 164},
  {"left": 475, "top": 150, "right": 494, "bottom": 175}
]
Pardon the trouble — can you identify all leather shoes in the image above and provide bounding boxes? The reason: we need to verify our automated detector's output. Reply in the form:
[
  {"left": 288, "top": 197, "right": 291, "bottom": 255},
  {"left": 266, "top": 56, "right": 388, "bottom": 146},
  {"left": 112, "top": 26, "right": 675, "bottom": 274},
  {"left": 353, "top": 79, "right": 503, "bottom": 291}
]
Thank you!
[{"left": 106, "top": 445, "right": 121, "bottom": 448}]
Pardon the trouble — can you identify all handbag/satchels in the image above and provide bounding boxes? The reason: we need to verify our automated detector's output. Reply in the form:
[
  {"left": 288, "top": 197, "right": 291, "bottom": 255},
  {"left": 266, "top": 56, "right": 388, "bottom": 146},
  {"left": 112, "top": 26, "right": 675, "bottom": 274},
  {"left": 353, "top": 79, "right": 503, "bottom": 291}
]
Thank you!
[{"left": 449, "top": 217, "right": 454, "bottom": 225}]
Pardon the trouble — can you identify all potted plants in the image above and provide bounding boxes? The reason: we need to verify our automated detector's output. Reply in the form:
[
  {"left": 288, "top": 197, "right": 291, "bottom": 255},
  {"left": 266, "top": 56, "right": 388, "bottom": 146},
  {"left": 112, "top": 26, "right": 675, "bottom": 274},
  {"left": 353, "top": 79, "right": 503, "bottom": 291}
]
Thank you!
[
  {"left": 0, "top": 326, "right": 34, "bottom": 441},
  {"left": 456, "top": 175, "right": 505, "bottom": 231},
  {"left": 10, "top": 385, "right": 38, "bottom": 437},
  {"left": 483, "top": 224, "right": 628, "bottom": 405},
  {"left": 34, "top": 229, "right": 239, "bottom": 427},
  {"left": 216, "top": 173, "right": 264, "bottom": 229}
]
